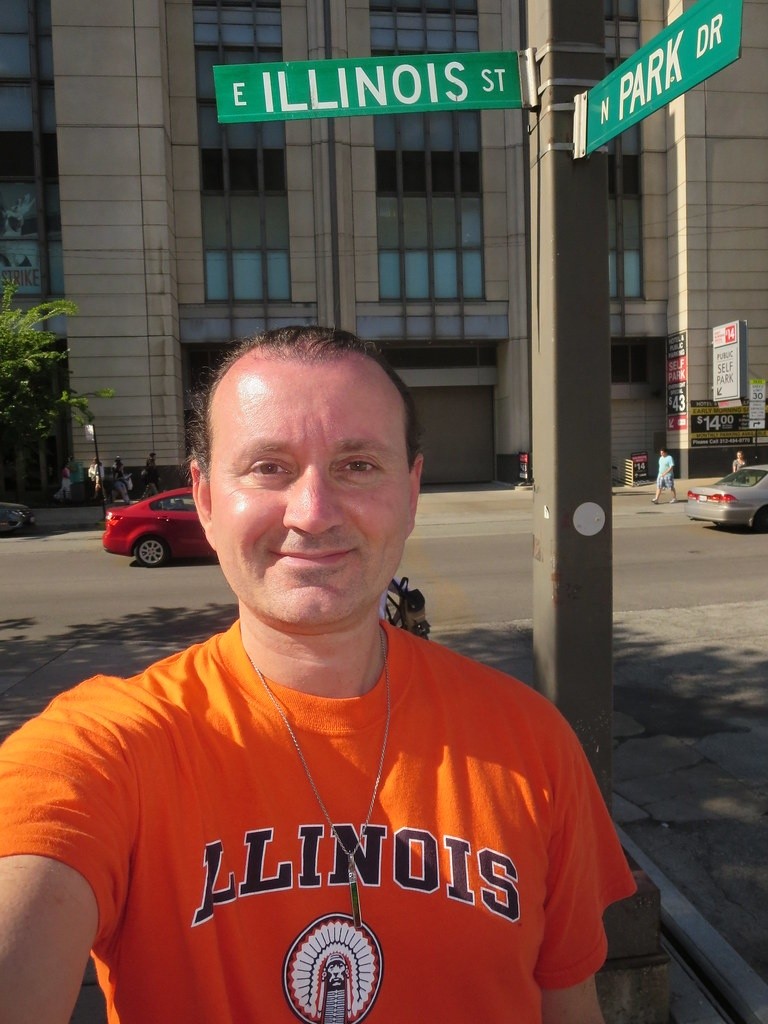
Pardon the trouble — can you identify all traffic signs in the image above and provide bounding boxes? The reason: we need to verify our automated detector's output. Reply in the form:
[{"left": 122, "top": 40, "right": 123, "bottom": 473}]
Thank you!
[
  {"left": 568, "top": 0, "right": 744, "bottom": 160},
  {"left": 214, "top": 50, "right": 523, "bottom": 124}
]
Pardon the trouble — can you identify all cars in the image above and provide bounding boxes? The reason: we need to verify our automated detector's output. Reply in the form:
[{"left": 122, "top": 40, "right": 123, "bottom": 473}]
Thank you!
[
  {"left": 686, "top": 463, "right": 768, "bottom": 532},
  {"left": 102, "top": 486, "right": 219, "bottom": 567},
  {"left": 0, "top": 502, "right": 38, "bottom": 535}
]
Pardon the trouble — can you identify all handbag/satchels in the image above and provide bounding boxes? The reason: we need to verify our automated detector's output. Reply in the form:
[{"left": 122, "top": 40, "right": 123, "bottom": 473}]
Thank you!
[{"left": 125, "top": 478, "right": 133, "bottom": 490}]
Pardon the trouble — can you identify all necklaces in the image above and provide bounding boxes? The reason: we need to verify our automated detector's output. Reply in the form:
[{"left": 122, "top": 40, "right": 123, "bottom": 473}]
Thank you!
[{"left": 252, "top": 627, "right": 391, "bottom": 930}]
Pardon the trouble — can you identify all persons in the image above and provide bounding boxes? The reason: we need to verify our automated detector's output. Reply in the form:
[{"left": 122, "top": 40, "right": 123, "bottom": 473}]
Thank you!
[
  {"left": 48, "top": 453, "right": 167, "bottom": 506},
  {"left": 0, "top": 323, "right": 636, "bottom": 1024},
  {"left": 733, "top": 451, "right": 747, "bottom": 472},
  {"left": 651, "top": 448, "right": 677, "bottom": 504}
]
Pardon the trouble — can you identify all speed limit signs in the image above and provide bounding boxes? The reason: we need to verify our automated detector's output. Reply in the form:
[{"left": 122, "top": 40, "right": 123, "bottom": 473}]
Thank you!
[{"left": 749, "top": 379, "right": 765, "bottom": 401}]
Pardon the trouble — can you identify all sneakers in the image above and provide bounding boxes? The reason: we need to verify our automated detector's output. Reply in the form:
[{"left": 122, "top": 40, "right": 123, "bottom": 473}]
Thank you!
[
  {"left": 652, "top": 499, "right": 658, "bottom": 504},
  {"left": 669, "top": 498, "right": 677, "bottom": 504}
]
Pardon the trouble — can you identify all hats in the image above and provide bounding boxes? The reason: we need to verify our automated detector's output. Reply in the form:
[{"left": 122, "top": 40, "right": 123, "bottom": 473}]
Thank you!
[{"left": 114, "top": 456, "right": 121, "bottom": 461}]
